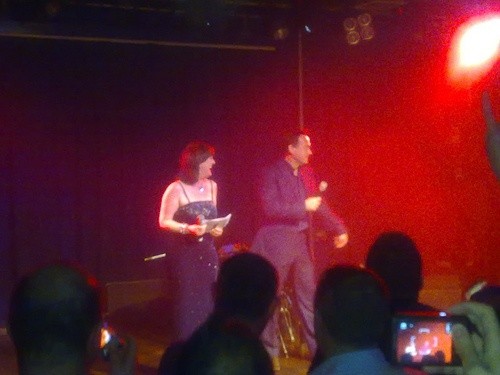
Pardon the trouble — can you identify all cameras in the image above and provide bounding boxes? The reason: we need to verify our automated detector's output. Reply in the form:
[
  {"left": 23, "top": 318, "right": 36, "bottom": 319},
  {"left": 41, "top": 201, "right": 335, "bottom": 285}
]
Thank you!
[
  {"left": 101, "top": 324, "right": 123, "bottom": 362},
  {"left": 391, "top": 311, "right": 470, "bottom": 366}
]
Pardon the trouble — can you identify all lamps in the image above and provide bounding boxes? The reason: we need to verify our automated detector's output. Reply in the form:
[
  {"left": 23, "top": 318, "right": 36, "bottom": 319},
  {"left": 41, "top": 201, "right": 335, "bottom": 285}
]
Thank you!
[{"left": 343, "top": 12, "right": 377, "bottom": 45}]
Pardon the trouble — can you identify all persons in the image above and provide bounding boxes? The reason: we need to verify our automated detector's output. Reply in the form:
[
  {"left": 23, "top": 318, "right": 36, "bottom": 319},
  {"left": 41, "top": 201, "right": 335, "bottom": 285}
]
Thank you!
[
  {"left": 244, "top": 127, "right": 350, "bottom": 370},
  {"left": 5, "top": 264, "right": 135, "bottom": 375},
  {"left": 158, "top": 252, "right": 280, "bottom": 375},
  {"left": 467, "top": 284, "right": 499, "bottom": 321},
  {"left": 422, "top": 299, "right": 500, "bottom": 374},
  {"left": 309, "top": 227, "right": 473, "bottom": 375},
  {"left": 307, "top": 264, "right": 407, "bottom": 374},
  {"left": 159, "top": 143, "right": 224, "bottom": 346}
]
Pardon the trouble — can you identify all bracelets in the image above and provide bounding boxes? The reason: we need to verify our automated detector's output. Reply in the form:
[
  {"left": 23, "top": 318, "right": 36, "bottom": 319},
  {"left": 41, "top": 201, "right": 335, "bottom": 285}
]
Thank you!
[{"left": 179, "top": 223, "right": 188, "bottom": 235}]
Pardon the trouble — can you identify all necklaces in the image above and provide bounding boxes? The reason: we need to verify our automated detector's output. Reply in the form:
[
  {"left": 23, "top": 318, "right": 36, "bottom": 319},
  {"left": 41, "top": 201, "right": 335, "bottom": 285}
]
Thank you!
[{"left": 194, "top": 180, "right": 205, "bottom": 193}]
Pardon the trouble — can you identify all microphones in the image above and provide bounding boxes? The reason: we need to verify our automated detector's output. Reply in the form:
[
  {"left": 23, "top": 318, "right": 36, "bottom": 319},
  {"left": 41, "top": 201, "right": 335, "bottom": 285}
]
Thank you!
[
  {"left": 309, "top": 180, "right": 328, "bottom": 217},
  {"left": 196, "top": 214, "right": 205, "bottom": 242}
]
